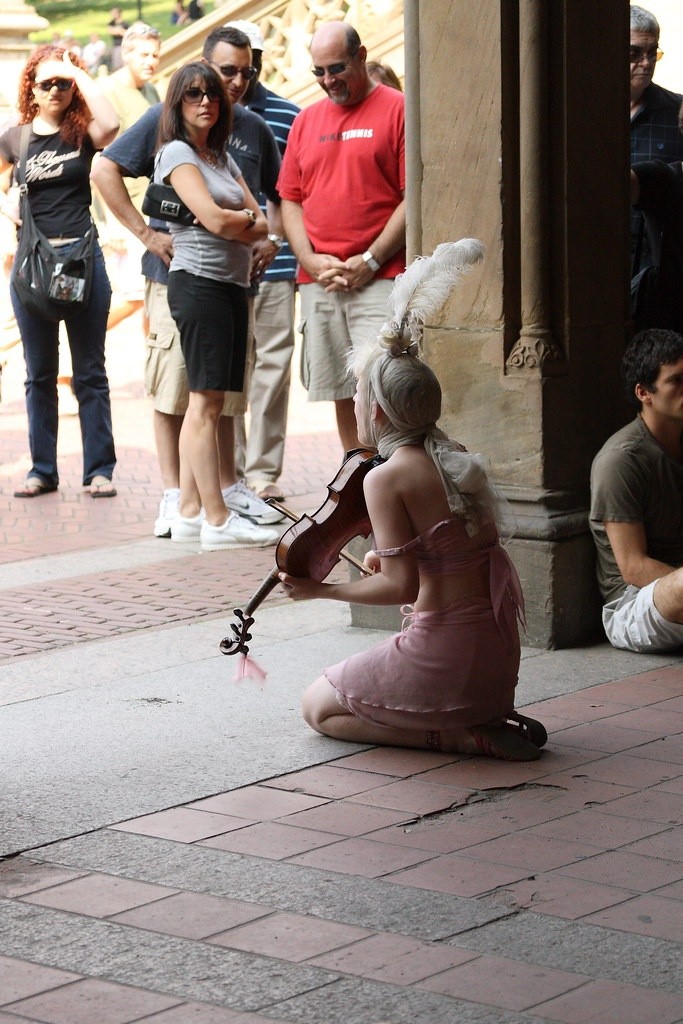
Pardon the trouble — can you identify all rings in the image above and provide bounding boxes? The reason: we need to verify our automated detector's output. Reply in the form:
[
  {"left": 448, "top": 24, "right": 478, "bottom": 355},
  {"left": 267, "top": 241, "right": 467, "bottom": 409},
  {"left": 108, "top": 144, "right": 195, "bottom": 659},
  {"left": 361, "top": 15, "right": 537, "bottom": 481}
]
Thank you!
[
  {"left": 258, "top": 260, "right": 264, "bottom": 266},
  {"left": 254, "top": 268, "right": 260, "bottom": 275}
]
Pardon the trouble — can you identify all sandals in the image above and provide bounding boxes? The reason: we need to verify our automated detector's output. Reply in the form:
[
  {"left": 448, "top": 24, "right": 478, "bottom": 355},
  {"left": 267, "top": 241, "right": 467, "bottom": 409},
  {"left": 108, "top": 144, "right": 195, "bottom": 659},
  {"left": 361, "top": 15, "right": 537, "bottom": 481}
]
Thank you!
[{"left": 247, "top": 479, "right": 286, "bottom": 501}]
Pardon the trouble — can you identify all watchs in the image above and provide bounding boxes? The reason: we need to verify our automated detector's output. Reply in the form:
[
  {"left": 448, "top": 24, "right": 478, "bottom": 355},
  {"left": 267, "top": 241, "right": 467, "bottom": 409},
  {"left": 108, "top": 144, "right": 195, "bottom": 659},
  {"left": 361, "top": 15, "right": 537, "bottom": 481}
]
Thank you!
[
  {"left": 267, "top": 235, "right": 282, "bottom": 251},
  {"left": 243, "top": 209, "right": 256, "bottom": 230}
]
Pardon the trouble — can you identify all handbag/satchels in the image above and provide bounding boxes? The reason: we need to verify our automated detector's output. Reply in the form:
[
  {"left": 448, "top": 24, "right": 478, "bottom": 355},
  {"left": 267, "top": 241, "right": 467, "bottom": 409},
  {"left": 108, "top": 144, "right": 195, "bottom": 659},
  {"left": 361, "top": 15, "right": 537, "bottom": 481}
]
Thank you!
[
  {"left": 140, "top": 183, "right": 201, "bottom": 227},
  {"left": 11, "top": 221, "right": 98, "bottom": 323}
]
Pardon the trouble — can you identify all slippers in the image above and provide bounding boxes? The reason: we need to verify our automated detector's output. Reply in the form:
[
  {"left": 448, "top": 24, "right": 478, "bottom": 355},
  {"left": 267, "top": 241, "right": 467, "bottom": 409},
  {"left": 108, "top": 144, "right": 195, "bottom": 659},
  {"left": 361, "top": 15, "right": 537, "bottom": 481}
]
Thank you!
[
  {"left": 14, "top": 476, "right": 58, "bottom": 498},
  {"left": 89, "top": 476, "right": 118, "bottom": 498}
]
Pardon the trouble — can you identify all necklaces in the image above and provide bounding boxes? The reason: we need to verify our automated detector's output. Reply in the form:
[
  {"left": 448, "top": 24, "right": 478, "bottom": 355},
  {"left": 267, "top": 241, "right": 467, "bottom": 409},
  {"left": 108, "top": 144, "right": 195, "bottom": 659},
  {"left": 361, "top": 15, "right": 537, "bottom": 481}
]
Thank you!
[{"left": 638, "top": 412, "right": 662, "bottom": 448}]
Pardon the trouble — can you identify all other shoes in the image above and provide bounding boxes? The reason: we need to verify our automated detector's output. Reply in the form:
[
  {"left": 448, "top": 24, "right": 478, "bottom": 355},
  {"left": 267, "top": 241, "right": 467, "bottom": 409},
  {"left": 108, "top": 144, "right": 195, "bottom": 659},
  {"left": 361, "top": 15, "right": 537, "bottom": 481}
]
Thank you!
[{"left": 458, "top": 708, "right": 548, "bottom": 762}]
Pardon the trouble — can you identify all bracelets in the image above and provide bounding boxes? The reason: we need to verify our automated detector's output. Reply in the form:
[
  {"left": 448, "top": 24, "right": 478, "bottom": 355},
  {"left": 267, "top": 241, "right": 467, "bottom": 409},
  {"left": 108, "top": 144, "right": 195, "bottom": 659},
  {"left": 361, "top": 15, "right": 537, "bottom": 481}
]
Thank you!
[{"left": 362, "top": 251, "right": 380, "bottom": 272}]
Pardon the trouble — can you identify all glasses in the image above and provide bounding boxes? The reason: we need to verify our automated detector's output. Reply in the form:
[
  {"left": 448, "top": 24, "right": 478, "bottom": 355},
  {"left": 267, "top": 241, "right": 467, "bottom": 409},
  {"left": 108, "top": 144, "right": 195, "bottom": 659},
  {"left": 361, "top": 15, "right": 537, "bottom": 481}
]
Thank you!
[
  {"left": 37, "top": 78, "right": 75, "bottom": 91},
  {"left": 209, "top": 61, "right": 258, "bottom": 81},
  {"left": 629, "top": 45, "right": 665, "bottom": 63},
  {"left": 182, "top": 88, "right": 223, "bottom": 103},
  {"left": 310, "top": 57, "right": 352, "bottom": 77},
  {"left": 124, "top": 26, "right": 160, "bottom": 37}
]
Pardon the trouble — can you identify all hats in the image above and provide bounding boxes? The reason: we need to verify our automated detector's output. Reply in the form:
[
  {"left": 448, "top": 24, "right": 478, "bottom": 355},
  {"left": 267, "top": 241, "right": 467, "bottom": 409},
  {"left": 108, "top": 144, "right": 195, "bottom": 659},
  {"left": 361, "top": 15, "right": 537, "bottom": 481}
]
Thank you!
[{"left": 224, "top": 19, "right": 266, "bottom": 52}]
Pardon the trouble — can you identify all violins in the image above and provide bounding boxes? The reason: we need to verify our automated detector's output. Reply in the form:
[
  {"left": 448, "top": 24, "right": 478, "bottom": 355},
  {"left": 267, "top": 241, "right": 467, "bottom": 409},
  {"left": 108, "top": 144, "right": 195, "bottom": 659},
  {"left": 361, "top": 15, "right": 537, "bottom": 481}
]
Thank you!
[{"left": 219, "top": 447, "right": 387, "bottom": 656}]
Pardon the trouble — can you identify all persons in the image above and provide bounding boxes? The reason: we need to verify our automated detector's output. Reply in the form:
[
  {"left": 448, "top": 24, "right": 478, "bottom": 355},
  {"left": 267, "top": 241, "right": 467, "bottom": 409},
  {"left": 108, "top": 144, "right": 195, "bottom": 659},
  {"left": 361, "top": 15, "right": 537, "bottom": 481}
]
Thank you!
[
  {"left": 58, "top": 21, "right": 160, "bottom": 385},
  {"left": 0, "top": 44, "right": 121, "bottom": 499},
  {"left": 276, "top": 26, "right": 407, "bottom": 454},
  {"left": 589, "top": 327, "right": 683, "bottom": 653},
  {"left": 171, "top": 0, "right": 205, "bottom": 28},
  {"left": 276, "top": 348, "right": 547, "bottom": 763},
  {"left": 623, "top": 5, "right": 683, "bottom": 336},
  {"left": 87, "top": 21, "right": 301, "bottom": 550},
  {"left": 53, "top": 5, "right": 131, "bottom": 75}
]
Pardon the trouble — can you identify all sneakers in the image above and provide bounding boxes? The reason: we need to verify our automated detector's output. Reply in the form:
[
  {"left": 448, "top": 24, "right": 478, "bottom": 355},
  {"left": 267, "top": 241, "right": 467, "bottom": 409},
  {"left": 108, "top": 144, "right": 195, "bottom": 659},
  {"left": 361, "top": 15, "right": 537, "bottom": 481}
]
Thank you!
[
  {"left": 170, "top": 509, "right": 205, "bottom": 543},
  {"left": 151, "top": 497, "right": 180, "bottom": 536},
  {"left": 222, "top": 478, "right": 286, "bottom": 525},
  {"left": 200, "top": 510, "right": 280, "bottom": 552}
]
